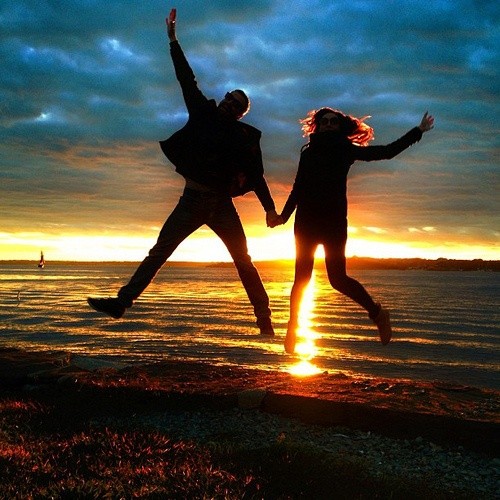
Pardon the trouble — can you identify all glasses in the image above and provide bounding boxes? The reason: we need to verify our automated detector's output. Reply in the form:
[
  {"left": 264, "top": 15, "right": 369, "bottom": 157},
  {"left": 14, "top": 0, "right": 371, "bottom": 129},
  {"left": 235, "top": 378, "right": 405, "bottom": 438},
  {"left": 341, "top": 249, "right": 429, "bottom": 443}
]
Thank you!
[
  {"left": 319, "top": 117, "right": 339, "bottom": 125},
  {"left": 225, "top": 92, "right": 243, "bottom": 108}
]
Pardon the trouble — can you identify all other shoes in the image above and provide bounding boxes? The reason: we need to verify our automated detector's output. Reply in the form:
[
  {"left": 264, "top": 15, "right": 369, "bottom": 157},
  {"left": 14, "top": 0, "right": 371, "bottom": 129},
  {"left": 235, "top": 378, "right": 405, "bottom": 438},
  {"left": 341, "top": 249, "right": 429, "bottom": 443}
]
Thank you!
[
  {"left": 375, "top": 310, "right": 393, "bottom": 346},
  {"left": 88, "top": 297, "right": 126, "bottom": 319},
  {"left": 284, "top": 324, "right": 298, "bottom": 354},
  {"left": 257, "top": 317, "right": 275, "bottom": 337}
]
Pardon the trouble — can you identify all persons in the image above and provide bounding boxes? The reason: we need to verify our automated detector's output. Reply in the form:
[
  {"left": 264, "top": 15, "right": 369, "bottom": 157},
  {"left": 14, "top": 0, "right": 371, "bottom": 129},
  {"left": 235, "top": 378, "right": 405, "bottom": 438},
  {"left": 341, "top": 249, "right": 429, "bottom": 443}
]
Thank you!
[
  {"left": 88, "top": 9, "right": 278, "bottom": 337},
  {"left": 268, "top": 108, "right": 436, "bottom": 356},
  {"left": 18, "top": 291, "right": 22, "bottom": 302}
]
